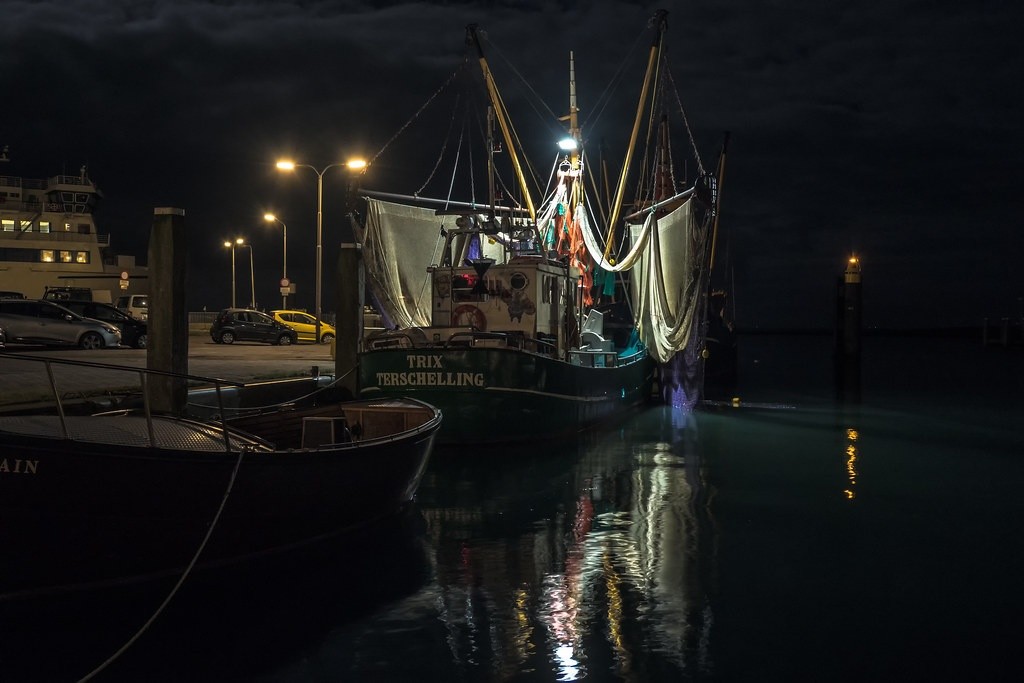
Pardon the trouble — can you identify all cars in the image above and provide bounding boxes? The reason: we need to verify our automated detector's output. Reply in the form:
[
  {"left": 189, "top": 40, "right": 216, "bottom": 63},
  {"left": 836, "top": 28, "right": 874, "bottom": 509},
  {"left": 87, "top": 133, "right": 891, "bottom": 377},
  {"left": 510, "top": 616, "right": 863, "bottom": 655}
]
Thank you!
[
  {"left": 210, "top": 309, "right": 298, "bottom": 346},
  {"left": 270, "top": 310, "right": 336, "bottom": 345},
  {"left": 45, "top": 299, "right": 148, "bottom": 349}
]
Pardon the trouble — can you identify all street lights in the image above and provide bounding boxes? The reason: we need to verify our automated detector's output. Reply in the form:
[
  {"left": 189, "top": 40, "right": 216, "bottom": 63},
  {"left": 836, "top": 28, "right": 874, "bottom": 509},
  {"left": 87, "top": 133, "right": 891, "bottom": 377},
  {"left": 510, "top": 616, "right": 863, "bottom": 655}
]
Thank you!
[
  {"left": 265, "top": 212, "right": 287, "bottom": 309},
  {"left": 277, "top": 157, "right": 365, "bottom": 344},
  {"left": 237, "top": 238, "right": 255, "bottom": 308},
  {"left": 225, "top": 241, "right": 235, "bottom": 308}
]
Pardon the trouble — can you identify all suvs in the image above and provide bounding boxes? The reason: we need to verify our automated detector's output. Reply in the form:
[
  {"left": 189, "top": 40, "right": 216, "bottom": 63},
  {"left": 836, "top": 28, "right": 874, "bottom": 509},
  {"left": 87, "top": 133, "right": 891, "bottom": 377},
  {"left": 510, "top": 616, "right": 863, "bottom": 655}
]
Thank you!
[{"left": 0, "top": 299, "right": 121, "bottom": 352}]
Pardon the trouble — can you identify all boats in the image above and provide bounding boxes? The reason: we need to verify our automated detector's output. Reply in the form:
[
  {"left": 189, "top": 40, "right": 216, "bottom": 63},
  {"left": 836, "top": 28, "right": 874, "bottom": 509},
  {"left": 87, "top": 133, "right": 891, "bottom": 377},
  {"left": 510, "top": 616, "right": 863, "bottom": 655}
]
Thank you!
[
  {"left": 0, "top": 145, "right": 150, "bottom": 302},
  {"left": 0, "top": 353, "right": 443, "bottom": 607}
]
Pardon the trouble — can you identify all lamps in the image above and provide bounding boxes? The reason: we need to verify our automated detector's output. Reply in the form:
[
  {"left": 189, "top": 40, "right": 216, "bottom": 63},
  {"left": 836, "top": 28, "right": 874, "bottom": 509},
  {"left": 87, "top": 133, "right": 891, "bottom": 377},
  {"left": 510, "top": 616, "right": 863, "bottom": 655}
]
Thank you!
[{"left": 483, "top": 221, "right": 499, "bottom": 235}]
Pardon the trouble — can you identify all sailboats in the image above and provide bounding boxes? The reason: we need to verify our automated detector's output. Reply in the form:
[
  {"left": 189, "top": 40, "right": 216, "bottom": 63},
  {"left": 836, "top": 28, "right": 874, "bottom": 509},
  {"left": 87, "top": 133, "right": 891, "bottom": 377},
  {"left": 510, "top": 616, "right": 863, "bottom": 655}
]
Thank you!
[{"left": 341, "top": 8, "right": 727, "bottom": 450}]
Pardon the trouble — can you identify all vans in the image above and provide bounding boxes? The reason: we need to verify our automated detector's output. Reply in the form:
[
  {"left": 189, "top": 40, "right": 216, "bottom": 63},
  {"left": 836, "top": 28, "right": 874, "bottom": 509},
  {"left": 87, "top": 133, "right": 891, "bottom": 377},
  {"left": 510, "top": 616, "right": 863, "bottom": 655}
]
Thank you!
[{"left": 112, "top": 294, "right": 148, "bottom": 320}]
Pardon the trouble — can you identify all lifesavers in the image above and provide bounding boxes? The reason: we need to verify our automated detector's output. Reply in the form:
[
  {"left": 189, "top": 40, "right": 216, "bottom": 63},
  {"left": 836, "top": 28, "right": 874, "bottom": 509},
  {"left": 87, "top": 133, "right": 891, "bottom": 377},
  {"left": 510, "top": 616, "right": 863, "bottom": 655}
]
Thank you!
[{"left": 448, "top": 303, "right": 487, "bottom": 332}]
